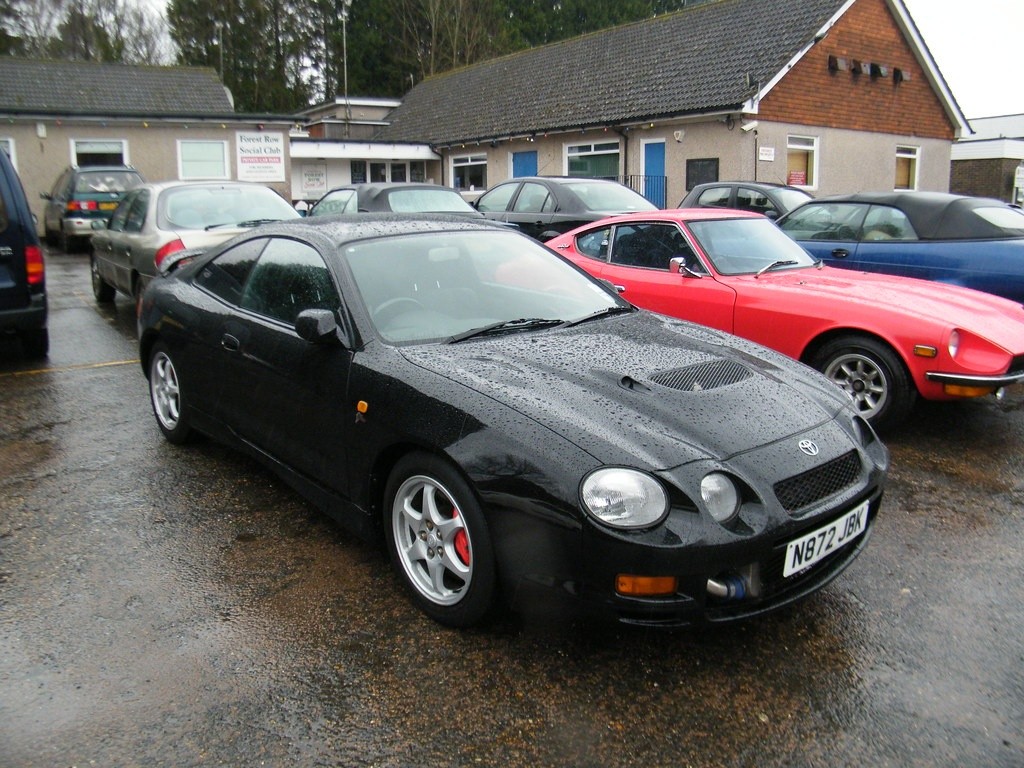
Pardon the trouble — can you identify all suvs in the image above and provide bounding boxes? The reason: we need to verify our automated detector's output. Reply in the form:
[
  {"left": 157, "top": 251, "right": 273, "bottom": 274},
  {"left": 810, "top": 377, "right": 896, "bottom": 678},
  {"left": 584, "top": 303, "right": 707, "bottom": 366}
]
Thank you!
[
  {"left": 40, "top": 165, "right": 146, "bottom": 255},
  {"left": 0, "top": 147, "right": 51, "bottom": 363}
]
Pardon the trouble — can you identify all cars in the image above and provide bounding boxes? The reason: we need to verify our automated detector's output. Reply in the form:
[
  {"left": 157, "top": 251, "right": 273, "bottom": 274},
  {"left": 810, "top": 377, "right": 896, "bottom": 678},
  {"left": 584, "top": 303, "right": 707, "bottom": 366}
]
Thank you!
[
  {"left": 469, "top": 177, "right": 663, "bottom": 245},
  {"left": 89, "top": 180, "right": 305, "bottom": 305},
  {"left": 773, "top": 189, "right": 1023, "bottom": 307},
  {"left": 674, "top": 181, "right": 836, "bottom": 229},
  {"left": 306, "top": 182, "right": 521, "bottom": 234}
]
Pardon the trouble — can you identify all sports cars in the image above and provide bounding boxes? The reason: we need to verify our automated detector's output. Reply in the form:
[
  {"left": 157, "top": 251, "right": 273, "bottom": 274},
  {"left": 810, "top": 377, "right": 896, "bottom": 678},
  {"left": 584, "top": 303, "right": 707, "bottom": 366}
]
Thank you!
[
  {"left": 137, "top": 210, "right": 891, "bottom": 639},
  {"left": 493, "top": 209, "right": 1024, "bottom": 438}
]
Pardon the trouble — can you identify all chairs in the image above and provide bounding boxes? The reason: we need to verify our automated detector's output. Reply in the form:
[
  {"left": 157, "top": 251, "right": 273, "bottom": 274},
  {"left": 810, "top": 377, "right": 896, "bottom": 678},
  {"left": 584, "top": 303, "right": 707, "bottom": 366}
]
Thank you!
[
  {"left": 737, "top": 193, "right": 751, "bottom": 210},
  {"left": 424, "top": 246, "right": 502, "bottom": 317},
  {"left": 755, "top": 193, "right": 767, "bottom": 214},
  {"left": 617, "top": 234, "right": 655, "bottom": 267},
  {"left": 673, "top": 233, "right": 705, "bottom": 267}
]
